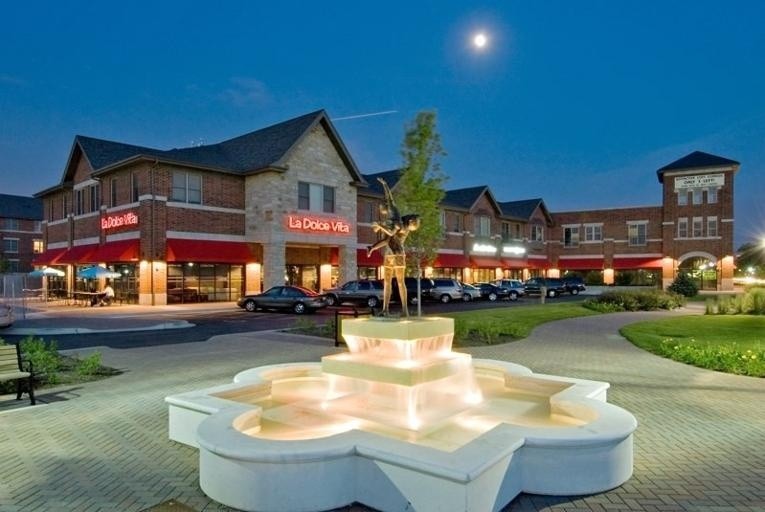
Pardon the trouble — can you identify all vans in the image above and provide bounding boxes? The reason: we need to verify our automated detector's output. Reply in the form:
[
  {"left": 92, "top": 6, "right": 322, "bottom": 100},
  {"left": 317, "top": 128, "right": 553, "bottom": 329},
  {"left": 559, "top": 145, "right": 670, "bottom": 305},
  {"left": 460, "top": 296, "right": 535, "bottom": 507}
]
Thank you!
[
  {"left": 426, "top": 276, "right": 463, "bottom": 303},
  {"left": 379, "top": 270, "right": 438, "bottom": 306}
]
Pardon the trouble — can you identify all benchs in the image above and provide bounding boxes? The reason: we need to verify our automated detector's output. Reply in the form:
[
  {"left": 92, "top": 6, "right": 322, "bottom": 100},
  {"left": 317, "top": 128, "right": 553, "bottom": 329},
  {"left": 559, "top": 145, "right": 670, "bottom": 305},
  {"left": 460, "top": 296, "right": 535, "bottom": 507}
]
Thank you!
[
  {"left": 187, "top": 286, "right": 237, "bottom": 301},
  {"left": 0, "top": 343, "right": 36, "bottom": 405}
]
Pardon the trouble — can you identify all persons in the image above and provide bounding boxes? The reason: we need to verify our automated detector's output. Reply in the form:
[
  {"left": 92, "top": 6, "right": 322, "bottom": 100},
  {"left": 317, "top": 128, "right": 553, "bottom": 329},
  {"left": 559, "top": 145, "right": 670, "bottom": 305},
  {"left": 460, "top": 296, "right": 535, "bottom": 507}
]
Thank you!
[
  {"left": 378, "top": 214, "right": 421, "bottom": 318},
  {"left": 367, "top": 176, "right": 401, "bottom": 260},
  {"left": 98, "top": 282, "right": 114, "bottom": 306}
]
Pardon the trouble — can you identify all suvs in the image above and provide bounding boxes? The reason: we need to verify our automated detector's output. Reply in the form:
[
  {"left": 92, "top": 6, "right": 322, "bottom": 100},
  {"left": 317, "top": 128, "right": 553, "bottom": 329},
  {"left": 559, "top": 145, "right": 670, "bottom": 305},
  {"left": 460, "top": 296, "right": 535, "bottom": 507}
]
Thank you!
[
  {"left": 498, "top": 279, "right": 525, "bottom": 302},
  {"left": 562, "top": 274, "right": 587, "bottom": 296},
  {"left": 522, "top": 277, "right": 567, "bottom": 296}
]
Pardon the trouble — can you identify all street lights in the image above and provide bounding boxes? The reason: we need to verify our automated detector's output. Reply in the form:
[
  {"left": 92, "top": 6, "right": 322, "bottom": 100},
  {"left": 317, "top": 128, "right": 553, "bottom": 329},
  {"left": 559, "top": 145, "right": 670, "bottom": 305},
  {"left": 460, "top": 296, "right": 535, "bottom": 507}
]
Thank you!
[{"left": 699, "top": 262, "right": 707, "bottom": 290}]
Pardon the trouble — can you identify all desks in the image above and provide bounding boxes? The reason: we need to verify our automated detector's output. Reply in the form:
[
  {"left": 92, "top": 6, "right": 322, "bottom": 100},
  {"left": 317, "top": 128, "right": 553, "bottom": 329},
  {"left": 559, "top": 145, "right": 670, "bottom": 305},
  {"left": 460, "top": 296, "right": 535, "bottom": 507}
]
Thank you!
[{"left": 71, "top": 291, "right": 99, "bottom": 307}]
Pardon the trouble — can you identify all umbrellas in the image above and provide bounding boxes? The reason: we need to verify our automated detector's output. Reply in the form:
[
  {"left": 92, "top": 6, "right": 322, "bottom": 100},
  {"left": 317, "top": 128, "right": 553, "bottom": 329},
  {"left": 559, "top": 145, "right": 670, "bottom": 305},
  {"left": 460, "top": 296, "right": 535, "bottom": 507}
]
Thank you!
[
  {"left": 76, "top": 265, "right": 122, "bottom": 280},
  {"left": 26, "top": 267, "right": 65, "bottom": 304}
]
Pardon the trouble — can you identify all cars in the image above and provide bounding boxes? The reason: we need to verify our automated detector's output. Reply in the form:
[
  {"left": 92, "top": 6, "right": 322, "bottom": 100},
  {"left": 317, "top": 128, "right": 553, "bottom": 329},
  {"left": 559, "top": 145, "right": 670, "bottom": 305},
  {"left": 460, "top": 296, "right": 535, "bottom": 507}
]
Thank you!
[
  {"left": 472, "top": 282, "right": 507, "bottom": 302},
  {"left": 457, "top": 280, "right": 484, "bottom": 302},
  {"left": 233, "top": 282, "right": 328, "bottom": 317},
  {"left": 324, "top": 274, "right": 391, "bottom": 310}
]
